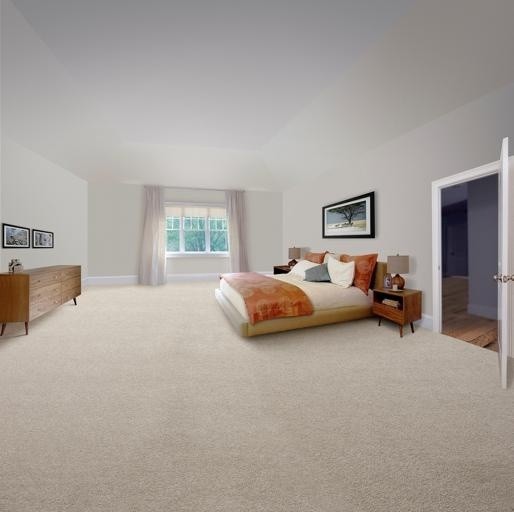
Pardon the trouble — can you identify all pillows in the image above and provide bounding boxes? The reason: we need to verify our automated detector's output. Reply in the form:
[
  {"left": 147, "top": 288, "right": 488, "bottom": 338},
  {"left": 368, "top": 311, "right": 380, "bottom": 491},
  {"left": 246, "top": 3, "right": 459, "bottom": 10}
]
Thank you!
[{"left": 290, "top": 251, "right": 378, "bottom": 296}]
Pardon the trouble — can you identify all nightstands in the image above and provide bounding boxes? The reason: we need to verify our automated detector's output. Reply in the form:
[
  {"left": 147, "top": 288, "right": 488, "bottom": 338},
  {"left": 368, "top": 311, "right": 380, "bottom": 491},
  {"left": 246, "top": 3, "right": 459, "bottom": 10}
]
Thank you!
[
  {"left": 273, "top": 265, "right": 291, "bottom": 274},
  {"left": 372, "top": 287, "right": 422, "bottom": 338}
]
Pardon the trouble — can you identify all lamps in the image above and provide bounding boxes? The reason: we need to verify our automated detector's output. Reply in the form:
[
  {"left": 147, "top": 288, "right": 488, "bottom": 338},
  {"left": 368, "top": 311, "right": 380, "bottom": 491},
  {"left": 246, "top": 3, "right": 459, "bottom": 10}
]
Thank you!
[
  {"left": 386, "top": 253, "right": 409, "bottom": 290},
  {"left": 287, "top": 245, "right": 302, "bottom": 268}
]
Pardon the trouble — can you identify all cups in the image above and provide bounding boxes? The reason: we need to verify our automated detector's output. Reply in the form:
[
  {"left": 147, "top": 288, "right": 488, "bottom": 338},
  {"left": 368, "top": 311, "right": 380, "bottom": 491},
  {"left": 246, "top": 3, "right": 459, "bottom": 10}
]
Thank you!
[{"left": 393, "top": 285, "right": 397, "bottom": 290}]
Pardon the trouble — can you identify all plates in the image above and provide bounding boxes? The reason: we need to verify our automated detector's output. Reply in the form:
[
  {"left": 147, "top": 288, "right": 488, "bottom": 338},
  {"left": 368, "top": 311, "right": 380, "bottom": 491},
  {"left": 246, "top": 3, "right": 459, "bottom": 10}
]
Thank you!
[{"left": 388, "top": 289, "right": 403, "bottom": 292}]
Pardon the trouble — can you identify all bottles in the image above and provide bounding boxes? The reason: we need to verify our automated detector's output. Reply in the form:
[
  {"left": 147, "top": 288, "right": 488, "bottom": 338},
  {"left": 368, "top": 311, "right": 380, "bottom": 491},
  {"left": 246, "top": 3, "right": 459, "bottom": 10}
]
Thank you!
[{"left": 382, "top": 273, "right": 391, "bottom": 289}]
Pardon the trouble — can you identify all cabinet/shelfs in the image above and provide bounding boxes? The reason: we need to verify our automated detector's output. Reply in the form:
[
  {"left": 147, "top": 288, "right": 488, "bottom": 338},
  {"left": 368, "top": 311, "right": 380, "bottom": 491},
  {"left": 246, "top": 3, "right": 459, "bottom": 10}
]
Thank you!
[{"left": 0, "top": 264, "right": 81, "bottom": 336}]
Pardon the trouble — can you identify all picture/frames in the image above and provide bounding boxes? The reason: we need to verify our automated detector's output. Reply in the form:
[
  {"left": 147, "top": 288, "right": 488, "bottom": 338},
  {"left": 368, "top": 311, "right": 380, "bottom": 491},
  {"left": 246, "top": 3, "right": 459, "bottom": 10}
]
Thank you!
[
  {"left": 321, "top": 191, "right": 375, "bottom": 239},
  {"left": 1, "top": 222, "right": 30, "bottom": 249},
  {"left": 31, "top": 228, "right": 54, "bottom": 249}
]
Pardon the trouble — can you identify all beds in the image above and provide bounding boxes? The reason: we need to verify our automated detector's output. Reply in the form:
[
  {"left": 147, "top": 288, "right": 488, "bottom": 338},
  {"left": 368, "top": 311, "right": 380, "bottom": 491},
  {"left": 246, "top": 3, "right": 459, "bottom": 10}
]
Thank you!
[{"left": 213, "top": 261, "right": 387, "bottom": 339}]
